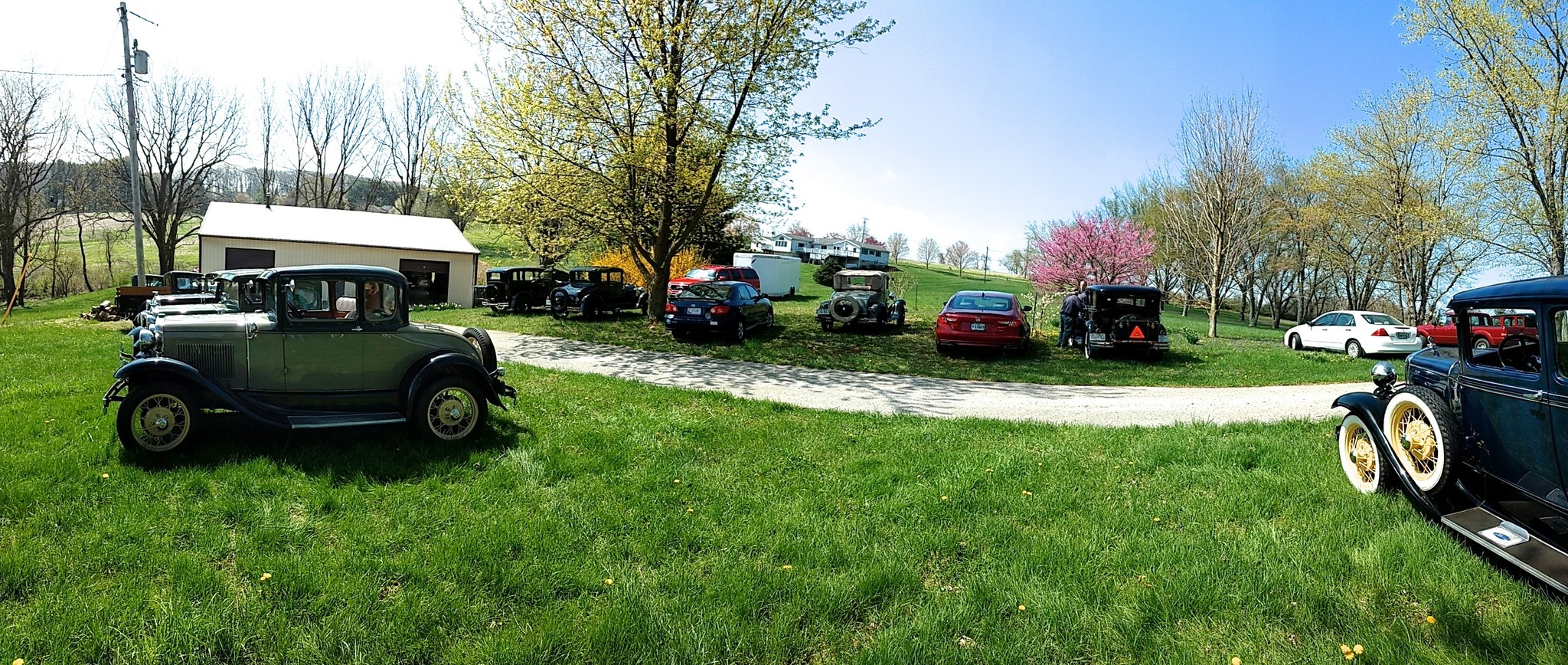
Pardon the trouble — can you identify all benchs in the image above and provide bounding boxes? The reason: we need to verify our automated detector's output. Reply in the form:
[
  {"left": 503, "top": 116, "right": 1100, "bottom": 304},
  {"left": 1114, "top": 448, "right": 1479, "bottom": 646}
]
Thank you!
[
  {"left": 345, "top": 309, "right": 378, "bottom": 318},
  {"left": 1367, "top": 319, "right": 1389, "bottom": 325},
  {"left": 960, "top": 303, "right": 1005, "bottom": 307}
]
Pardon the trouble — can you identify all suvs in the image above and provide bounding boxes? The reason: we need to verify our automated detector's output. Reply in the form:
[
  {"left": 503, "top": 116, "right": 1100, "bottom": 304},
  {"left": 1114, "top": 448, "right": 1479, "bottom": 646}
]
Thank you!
[
  {"left": 102, "top": 269, "right": 517, "bottom": 462},
  {"left": 550, "top": 267, "right": 647, "bottom": 319},
  {"left": 473, "top": 267, "right": 569, "bottom": 315},
  {"left": 1330, "top": 273, "right": 1568, "bottom": 596},
  {"left": 667, "top": 264, "right": 761, "bottom": 299},
  {"left": 1069, "top": 285, "right": 1169, "bottom": 362},
  {"left": 1489, "top": 314, "right": 1539, "bottom": 339}
]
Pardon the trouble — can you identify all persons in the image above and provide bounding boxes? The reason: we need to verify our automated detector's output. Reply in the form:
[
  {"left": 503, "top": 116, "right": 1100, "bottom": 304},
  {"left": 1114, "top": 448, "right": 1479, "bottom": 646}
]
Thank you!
[
  {"left": 1072, "top": 279, "right": 1091, "bottom": 348},
  {"left": 1056, "top": 287, "right": 1086, "bottom": 349}
]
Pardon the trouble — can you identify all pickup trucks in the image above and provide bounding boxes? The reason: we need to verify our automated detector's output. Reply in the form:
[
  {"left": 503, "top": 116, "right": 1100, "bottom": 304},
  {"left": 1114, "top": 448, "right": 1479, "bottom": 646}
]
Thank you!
[{"left": 1411, "top": 310, "right": 1524, "bottom": 349}]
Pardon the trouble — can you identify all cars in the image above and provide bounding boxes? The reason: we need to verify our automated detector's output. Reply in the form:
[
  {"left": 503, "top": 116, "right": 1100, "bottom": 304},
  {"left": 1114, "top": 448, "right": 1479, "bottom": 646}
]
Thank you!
[
  {"left": 936, "top": 291, "right": 1032, "bottom": 356},
  {"left": 816, "top": 269, "right": 907, "bottom": 332},
  {"left": 665, "top": 280, "right": 774, "bottom": 342},
  {"left": 1284, "top": 310, "right": 1422, "bottom": 359}
]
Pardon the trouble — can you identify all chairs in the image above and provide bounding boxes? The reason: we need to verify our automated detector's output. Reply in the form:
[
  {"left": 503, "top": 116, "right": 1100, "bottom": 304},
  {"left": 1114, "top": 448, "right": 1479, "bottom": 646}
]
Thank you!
[
  {"left": 964, "top": 299, "right": 975, "bottom": 304},
  {"left": 1339, "top": 316, "right": 1348, "bottom": 326}
]
